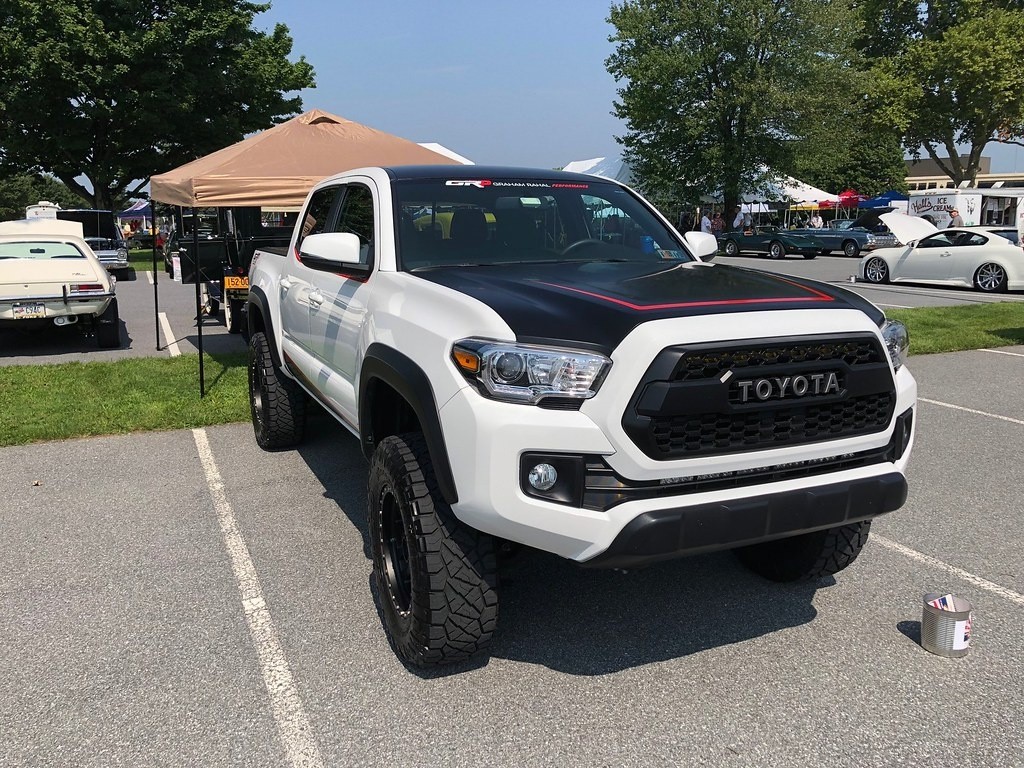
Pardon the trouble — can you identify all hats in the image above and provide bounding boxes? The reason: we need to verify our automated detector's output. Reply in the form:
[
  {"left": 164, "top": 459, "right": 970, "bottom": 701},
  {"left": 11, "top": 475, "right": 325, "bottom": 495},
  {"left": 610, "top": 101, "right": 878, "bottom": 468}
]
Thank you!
[
  {"left": 703, "top": 208, "right": 710, "bottom": 214},
  {"left": 946, "top": 205, "right": 958, "bottom": 212}
]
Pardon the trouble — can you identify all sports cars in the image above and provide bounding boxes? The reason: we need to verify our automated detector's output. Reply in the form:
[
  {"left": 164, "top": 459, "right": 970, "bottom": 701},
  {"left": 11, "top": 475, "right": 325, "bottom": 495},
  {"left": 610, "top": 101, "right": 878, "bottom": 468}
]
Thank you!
[
  {"left": 787, "top": 204, "right": 900, "bottom": 258},
  {"left": 858, "top": 213, "right": 1024, "bottom": 294},
  {"left": 716, "top": 224, "right": 826, "bottom": 260}
]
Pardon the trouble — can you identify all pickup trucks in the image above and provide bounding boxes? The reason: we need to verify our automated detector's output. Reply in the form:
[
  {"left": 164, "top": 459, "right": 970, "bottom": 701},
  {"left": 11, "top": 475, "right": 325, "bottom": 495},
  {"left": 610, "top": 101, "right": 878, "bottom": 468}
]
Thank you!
[{"left": 234, "top": 164, "right": 921, "bottom": 672}]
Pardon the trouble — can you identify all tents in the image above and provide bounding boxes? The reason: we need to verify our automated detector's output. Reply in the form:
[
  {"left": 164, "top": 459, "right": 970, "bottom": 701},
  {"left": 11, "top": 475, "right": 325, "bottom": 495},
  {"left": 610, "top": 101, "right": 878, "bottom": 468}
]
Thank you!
[
  {"left": 147, "top": 108, "right": 465, "bottom": 399},
  {"left": 559, "top": 155, "right": 909, "bottom": 228}
]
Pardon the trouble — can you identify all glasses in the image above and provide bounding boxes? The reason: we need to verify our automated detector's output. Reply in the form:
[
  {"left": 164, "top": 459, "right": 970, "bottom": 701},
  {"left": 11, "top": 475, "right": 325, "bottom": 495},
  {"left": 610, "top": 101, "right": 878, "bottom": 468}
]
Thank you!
[{"left": 716, "top": 214, "right": 719, "bottom": 216}]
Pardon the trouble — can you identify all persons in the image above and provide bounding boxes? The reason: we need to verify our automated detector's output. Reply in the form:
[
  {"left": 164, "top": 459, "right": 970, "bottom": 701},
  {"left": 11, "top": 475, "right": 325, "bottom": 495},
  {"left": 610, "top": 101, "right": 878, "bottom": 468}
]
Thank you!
[
  {"left": 945, "top": 206, "right": 964, "bottom": 244},
  {"left": 699, "top": 205, "right": 823, "bottom": 253}
]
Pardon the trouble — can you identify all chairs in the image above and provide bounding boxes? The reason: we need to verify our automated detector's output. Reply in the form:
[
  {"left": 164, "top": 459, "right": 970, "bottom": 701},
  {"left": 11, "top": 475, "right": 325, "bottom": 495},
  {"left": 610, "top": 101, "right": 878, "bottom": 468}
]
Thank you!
[{"left": 402, "top": 206, "right": 541, "bottom": 262}]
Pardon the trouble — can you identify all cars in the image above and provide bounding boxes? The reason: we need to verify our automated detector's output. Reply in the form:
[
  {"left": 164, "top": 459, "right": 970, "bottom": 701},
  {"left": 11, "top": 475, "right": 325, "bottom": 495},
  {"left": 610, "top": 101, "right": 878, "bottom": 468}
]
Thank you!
[
  {"left": 160, "top": 203, "right": 307, "bottom": 346},
  {"left": 55, "top": 207, "right": 130, "bottom": 282},
  {"left": 411, "top": 205, "right": 498, "bottom": 241},
  {"left": 0, "top": 217, "right": 122, "bottom": 350}
]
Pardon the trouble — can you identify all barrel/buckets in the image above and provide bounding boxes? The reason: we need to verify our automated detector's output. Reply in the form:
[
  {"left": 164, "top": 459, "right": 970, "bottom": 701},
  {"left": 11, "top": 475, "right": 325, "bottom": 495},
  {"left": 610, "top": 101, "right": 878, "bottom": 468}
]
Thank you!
[
  {"left": 849, "top": 274, "right": 857, "bottom": 283},
  {"left": 640, "top": 236, "right": 654, "bottom": 254}
]
